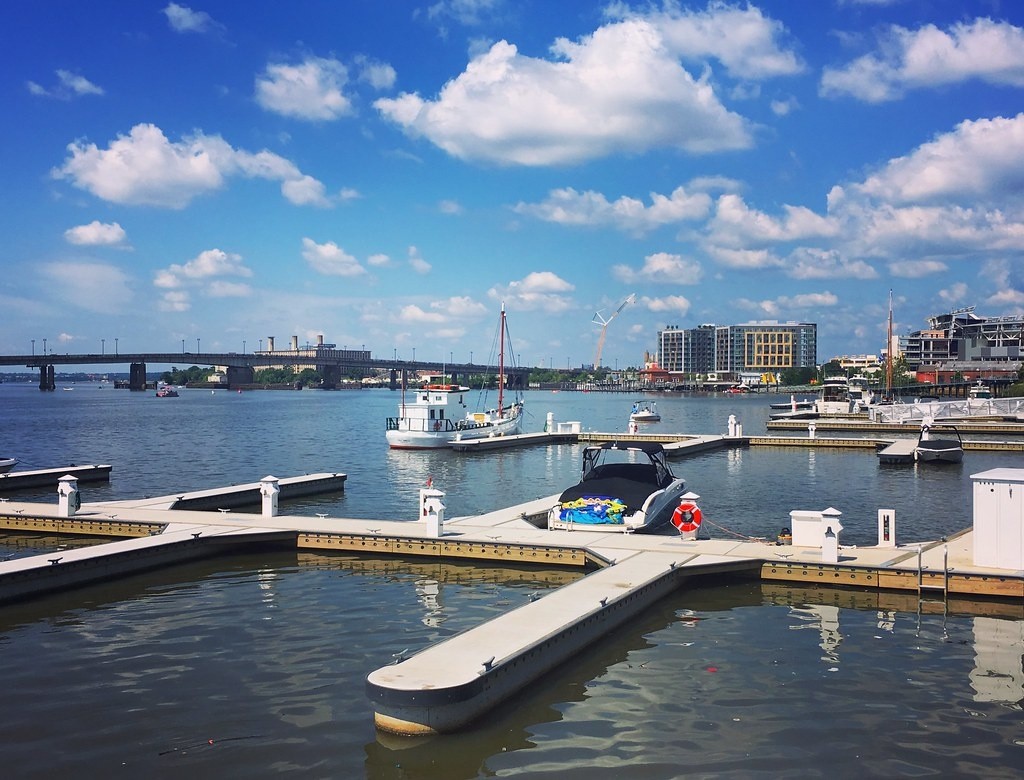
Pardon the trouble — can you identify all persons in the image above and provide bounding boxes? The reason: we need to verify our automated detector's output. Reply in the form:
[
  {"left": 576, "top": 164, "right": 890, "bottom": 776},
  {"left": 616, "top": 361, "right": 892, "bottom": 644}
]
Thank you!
[
  {"left": 644, "top": 407, "right": 649, "bottom": 411},
  {"left": 632, "top": 405, "right": 637, "bottom": 414}
]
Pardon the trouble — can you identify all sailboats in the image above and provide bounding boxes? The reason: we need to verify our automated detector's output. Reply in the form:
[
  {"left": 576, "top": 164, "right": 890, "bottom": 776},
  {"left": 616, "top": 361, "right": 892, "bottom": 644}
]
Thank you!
[{"left": 387, "top": 297, "right": 535, "bottom": 450}]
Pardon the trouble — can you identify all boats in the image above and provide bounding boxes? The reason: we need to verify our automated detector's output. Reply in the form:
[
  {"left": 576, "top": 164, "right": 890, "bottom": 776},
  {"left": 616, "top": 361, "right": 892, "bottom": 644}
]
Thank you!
[
  {"left": 876, "top": 416, "right": 964, "bottom": 464},
  {"left": 63, "top": 387, "right": 75, "bottom": 391},
  {"left": 769, "top": 401, "right": 816, "bottom": 410},
  {"left": 547, "top": 441, "right": 688, "bottom": 535},
  {"left": 156, "top": 385, "right": 179, "bottom": 398},
  {"left": 768, "top": 410, "right": 820, "bottom": 420},
  {"left": 815, "top": 373, "right": 998, "bottom": 416},
  {"left": 413, "top": 384, "right": 472, "bottom": 393},
  {"left": 0, "top": 458, "right": 18, "bottom": 473},
  {"left": 629, "top": 399, "right": 661, "bottom": 421}
]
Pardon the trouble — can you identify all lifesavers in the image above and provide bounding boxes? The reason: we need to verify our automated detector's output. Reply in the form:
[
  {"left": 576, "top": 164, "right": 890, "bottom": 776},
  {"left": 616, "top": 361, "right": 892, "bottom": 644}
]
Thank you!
[
  {"left": 433, "top": 423, "right": 441, "bottom": 431},
  {"left": 672, "top": 504, "right": 702, "bottom": 532}
]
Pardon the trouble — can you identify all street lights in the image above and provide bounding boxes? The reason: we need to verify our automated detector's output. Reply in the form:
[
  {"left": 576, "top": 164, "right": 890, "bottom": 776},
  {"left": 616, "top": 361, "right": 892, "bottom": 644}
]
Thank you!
[
  {"left": 197, "top": 338, "right": 201, "bottom": 355},
  {"left": 43, "top": 338, "right": 47, "bottom": 356},
  {"left": 616, "top": 359, "right": 618, "bottom": 371},
  {"left": 259, "top": 340, "right": 262, "bottom": 353},
  {"left": 243, "top": 340, "right": 246, "bottom": 355},
  {"left": 568, "top": 357, "right": 570, "bottom": 369},
  {"left": 115, "top": 338, "right": 119, "bottom": 354},
  {"left": 451, "top": 352, "right": 453, "bottom": 364},
  {"left": 412, "top": 347, "right": 415, "bottom": 362},
  {"left": 470, "top": 351, "right": 473, "bottom": 364},
  {"left": 395, "top": 349, "right": 396, "bottom": 360},
  {"left": 344, "top": 346, "right": 347, "bottom": 357},
  {"left": 182, "top": 339, "right": 185, "bottom": 354},
  {"left": 550, "top": 357, "right": 552, "bottom": 368},
  {"left": 362, "top": 345, "right": 365, "bottom": 360},
  {"left": 518, "top": 354, "right": 520, "bottom": 367},
  {"left": 600, "top": 358, "right": 602, "bottom": 370},
  {"left": 31, "top": 340, "right": 36, "bottom": 356},
  {"left": 101, "top": 339, "right": 105, "bottom": 354}
]
